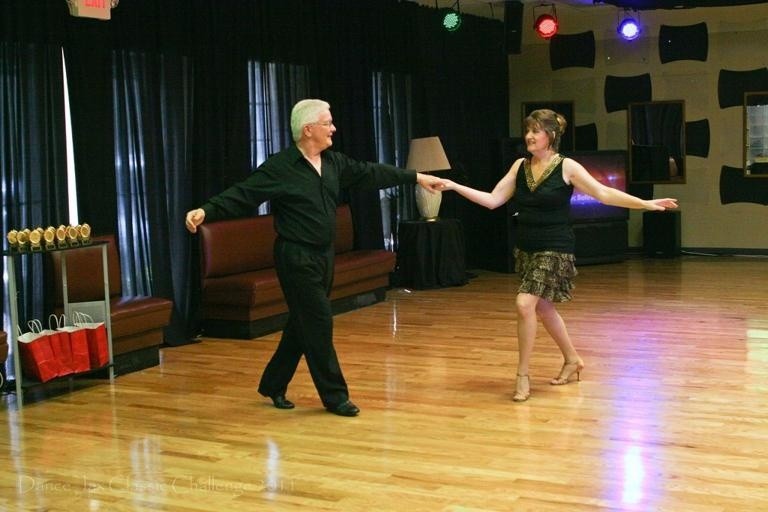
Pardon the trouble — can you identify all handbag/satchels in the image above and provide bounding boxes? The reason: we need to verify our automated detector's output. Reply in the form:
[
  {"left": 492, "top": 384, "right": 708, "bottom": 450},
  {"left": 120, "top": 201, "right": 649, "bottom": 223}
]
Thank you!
[{"left": 20, "top": 312, "right": 109, "bottom": 385}]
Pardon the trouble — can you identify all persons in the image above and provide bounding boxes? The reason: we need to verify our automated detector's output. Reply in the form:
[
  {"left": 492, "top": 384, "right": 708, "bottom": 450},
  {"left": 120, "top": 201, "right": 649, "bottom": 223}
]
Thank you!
[
  {"left": 430, "top": 109, "right": 679, "bottom": 404},
  {"left": 183, "top": 98, "right": 444, "bottom": 418}
]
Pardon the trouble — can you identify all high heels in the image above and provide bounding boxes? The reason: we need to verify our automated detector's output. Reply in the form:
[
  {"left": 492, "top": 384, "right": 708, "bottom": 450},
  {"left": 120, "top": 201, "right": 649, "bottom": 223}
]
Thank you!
[
  {"left": 513, "top": 373, "right": 531, "bottom": 402},
  {"left": 551, "top": 357, "right": 584, "bottom": 385}
]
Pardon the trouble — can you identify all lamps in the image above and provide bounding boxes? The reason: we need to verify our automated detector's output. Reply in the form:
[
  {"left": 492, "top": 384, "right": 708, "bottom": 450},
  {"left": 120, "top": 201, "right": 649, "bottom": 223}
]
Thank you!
[
  {"left": 405, "top": 135, "right": 451, "bottom": 223},
  {"left": 432, "top": 0, "right": 642, "bottom": 41}
]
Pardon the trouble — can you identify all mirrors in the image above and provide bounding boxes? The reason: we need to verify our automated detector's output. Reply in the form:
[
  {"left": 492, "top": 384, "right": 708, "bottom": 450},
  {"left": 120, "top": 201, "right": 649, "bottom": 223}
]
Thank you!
[
  {"left": 626, "top": 98, "right": 687, "bottom": 185},
  {"left": 521, "top": 99, "right": 576, "bottom": 154},
  {"left": 742, "top": 90, "right": 767, "bottom": 178}
]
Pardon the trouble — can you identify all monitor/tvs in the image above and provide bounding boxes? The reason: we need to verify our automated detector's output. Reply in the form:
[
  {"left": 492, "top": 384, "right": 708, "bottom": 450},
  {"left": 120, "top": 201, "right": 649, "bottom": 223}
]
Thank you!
[{"left": 570, "top": 149, "right": 630, "bottom": 222}]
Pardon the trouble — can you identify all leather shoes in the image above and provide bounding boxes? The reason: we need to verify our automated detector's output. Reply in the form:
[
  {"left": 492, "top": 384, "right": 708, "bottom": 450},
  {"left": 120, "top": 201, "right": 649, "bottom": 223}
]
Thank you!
[
  {"left": 258, "top": 386, "right": 294, "bottom": 408},
  {"left": 329, "top": 399, "right": 359, "bottom": 416}
]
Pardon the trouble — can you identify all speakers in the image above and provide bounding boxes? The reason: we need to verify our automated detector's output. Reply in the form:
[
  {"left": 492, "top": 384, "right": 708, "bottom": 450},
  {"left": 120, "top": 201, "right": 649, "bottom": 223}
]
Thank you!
[{"left": 642, "top": 210, "right": 681, "bottom": 259}]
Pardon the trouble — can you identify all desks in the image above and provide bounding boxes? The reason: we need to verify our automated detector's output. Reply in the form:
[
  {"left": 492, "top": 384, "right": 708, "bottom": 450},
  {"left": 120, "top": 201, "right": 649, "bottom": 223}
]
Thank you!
[
  {"left": 398, "top": 215, "right": 477, "bottom": 290},
  {"left": 2, "top": 239, "right": 116, "bottom": 411}
]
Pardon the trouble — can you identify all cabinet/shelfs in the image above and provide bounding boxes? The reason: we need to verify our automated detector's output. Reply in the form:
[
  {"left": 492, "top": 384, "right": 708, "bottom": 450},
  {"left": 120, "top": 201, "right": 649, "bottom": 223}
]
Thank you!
[{"left": 502, "top": 219, "right": 628, "bottom": 273}]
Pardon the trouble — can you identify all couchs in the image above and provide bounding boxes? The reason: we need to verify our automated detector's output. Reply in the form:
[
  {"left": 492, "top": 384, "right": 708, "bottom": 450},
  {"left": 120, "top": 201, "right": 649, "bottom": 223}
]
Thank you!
[
  {"left": 42, "top": 237, "right": 174, "bottom": 378},
  {"left": 192, "top": 205, "right": 398, "bottom": 340}
]
